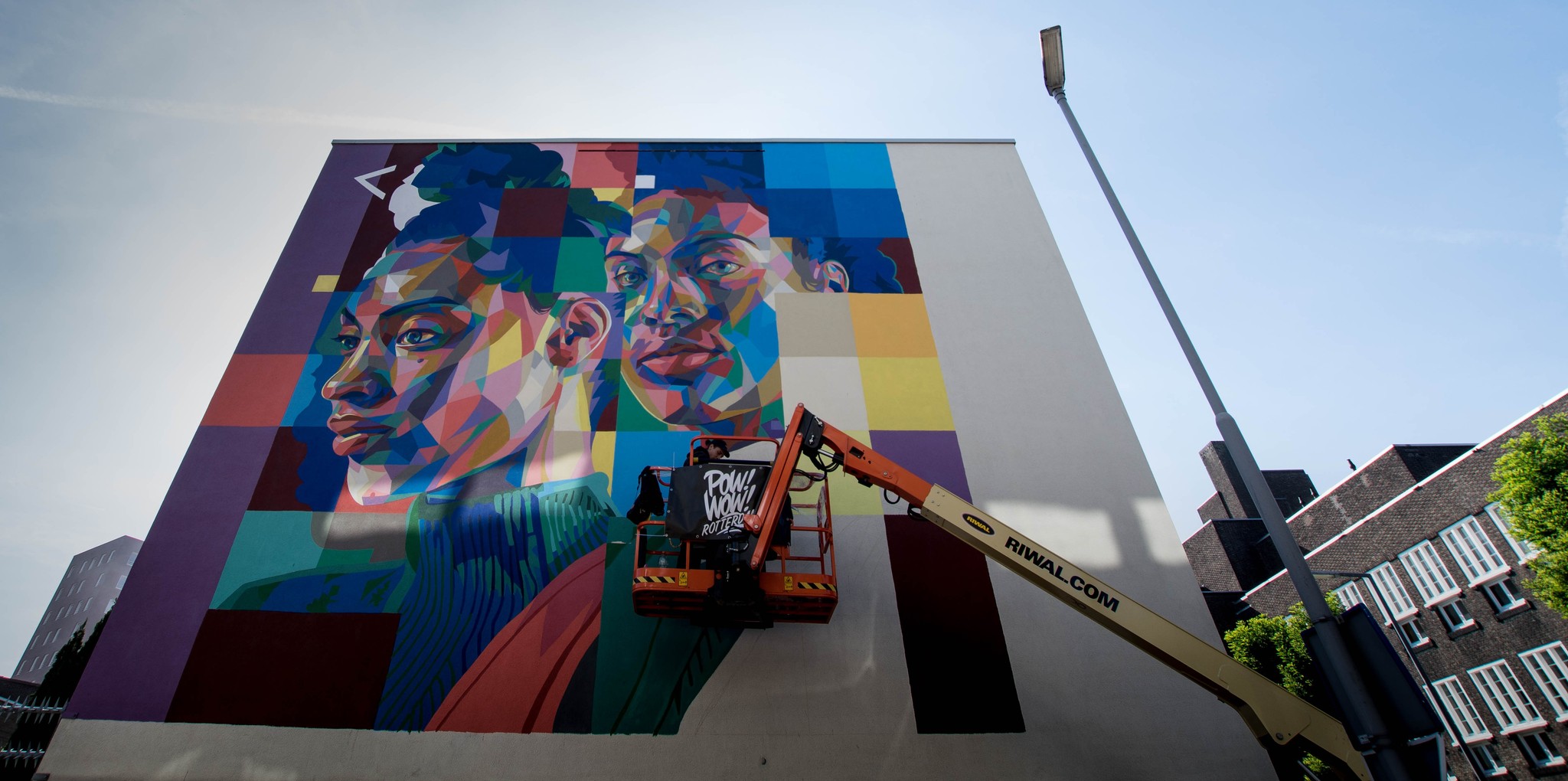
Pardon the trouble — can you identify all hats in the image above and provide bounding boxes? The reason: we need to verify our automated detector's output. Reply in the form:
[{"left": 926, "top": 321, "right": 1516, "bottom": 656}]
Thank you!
[{"left": 710, "top": 439, "right": 730, "bottom": 458}]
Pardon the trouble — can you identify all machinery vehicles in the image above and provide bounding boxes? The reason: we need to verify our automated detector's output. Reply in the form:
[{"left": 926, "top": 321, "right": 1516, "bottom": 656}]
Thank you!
[{"left": 630, "top": 402, "right": 1376, "bottom": 781}]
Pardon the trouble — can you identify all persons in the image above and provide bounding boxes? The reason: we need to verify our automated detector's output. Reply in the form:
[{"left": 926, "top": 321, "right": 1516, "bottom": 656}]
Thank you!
[{"left": 674, "top": 439, "right": 731, "bottom": 568}]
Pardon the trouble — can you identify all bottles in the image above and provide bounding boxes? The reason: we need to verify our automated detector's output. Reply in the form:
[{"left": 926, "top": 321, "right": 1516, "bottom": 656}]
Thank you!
[{"left": 659, "top": 554, "right": 668, "bottom": 568}]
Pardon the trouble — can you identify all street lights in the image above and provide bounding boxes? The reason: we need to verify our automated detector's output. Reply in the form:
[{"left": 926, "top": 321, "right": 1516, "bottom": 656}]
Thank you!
[{"left": 1035, "top": 20, "right": 1405, "bottom": 781}]
